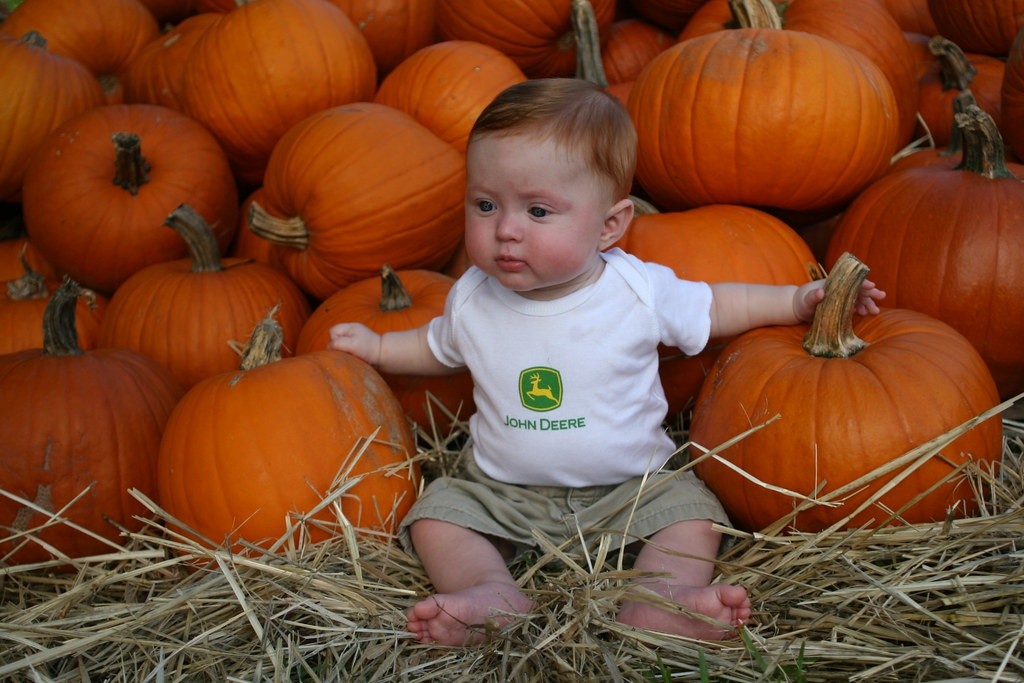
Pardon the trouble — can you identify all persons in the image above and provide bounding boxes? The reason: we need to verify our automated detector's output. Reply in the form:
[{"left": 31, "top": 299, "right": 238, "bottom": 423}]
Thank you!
[{"left": 327, "top": 79, "right": 887, "bottom": 645}]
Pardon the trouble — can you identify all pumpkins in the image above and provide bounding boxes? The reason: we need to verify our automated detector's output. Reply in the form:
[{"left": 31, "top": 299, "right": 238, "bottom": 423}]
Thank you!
[{"left": 1, "top": 0, "right": 1024, "bottom": 569}]
